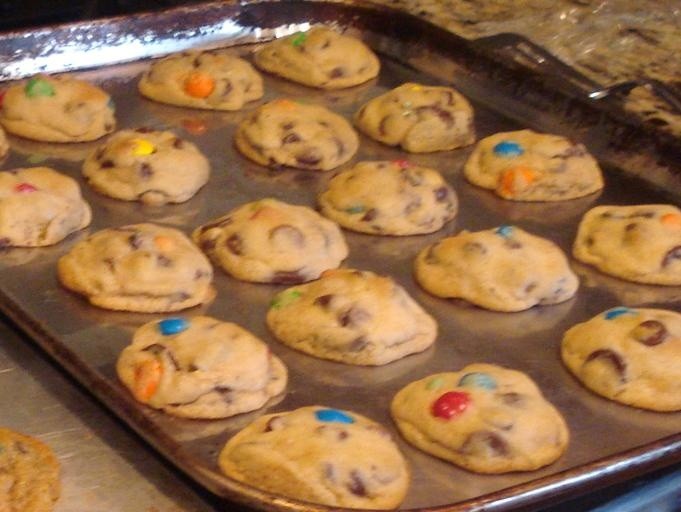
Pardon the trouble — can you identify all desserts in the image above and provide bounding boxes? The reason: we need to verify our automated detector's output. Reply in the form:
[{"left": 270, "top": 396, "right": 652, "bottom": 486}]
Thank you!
[{"left": 1, "top": 22, "right": 680, "bottom": 511}]
